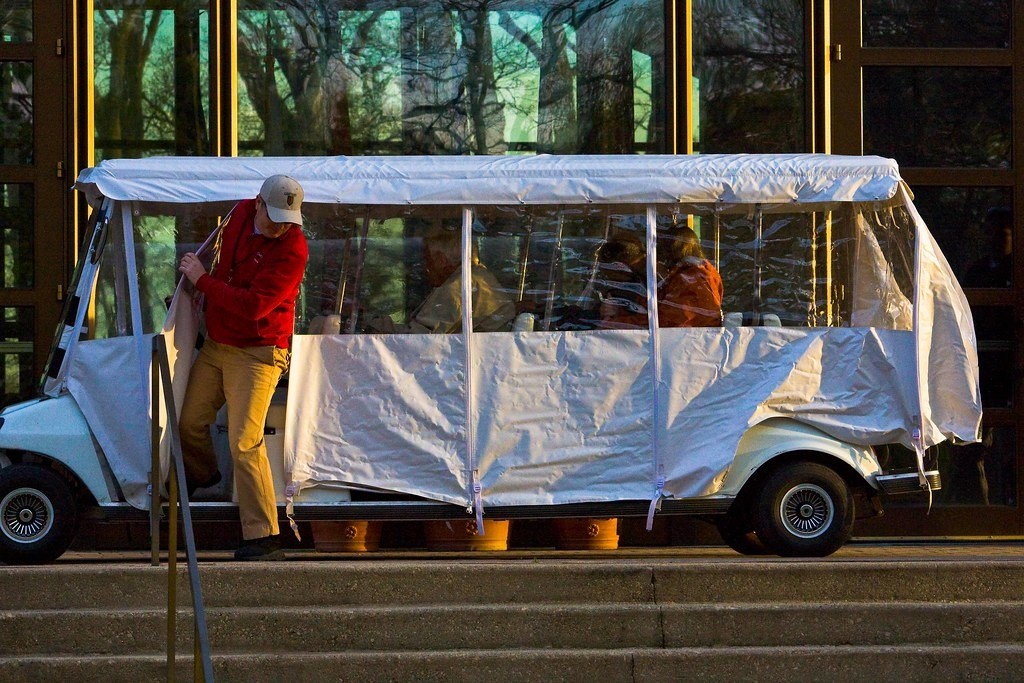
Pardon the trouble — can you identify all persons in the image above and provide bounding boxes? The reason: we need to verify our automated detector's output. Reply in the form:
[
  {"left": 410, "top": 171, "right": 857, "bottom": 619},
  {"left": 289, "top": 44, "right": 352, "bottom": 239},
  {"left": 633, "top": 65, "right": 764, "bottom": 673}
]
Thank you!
[
  {"left": 944, "top": 207, "right": 1014, "bottom": 500},
  {"left": 159, "top": 175, "right": 310, "bottom": 561},
  {"left": 307, "top": 211, "right": 726, "bottom": 335}
]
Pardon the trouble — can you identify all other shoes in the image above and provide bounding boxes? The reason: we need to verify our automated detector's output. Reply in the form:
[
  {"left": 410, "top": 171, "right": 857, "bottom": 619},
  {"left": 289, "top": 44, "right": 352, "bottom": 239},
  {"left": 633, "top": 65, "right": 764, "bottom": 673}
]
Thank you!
[
  {"left": 224, "top": 538, "right": 286, "bottom": 564},
  {"left": 147, "top": 469, "right": 222, "bottom": 502}
]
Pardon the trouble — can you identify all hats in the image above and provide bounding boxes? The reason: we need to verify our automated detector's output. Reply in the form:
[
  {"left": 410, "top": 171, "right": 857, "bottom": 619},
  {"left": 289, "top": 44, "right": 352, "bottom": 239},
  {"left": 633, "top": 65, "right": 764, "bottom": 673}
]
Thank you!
[
  {"left": 599, "top": 243, "right": 626, "bottom": 262},
  {"left": 260, "top": 174, "right": 304, "bottom": 226},
  {"left": 614, "top": 232, "right": 645, "bottom": 251}
]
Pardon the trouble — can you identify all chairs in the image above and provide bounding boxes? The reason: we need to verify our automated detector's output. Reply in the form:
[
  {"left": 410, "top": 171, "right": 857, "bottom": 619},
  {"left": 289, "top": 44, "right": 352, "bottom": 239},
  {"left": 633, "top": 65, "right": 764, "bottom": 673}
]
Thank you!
[{"left": 215, "top": 313, "right": 781, "bottom": 504}]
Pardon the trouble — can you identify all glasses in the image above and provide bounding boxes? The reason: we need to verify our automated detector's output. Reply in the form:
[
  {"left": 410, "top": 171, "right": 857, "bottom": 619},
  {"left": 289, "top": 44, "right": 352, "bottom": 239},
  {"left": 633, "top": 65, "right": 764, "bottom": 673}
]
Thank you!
[{"left": 441, "top": 218, "right": 458, "bottom": 231}]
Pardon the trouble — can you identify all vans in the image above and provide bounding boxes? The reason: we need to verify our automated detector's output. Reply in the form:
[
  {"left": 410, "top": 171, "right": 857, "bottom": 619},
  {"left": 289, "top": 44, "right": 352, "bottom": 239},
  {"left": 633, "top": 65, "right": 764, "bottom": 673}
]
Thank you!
[{"left": 0, "top": 154, "right": 986, "bottom": 567}]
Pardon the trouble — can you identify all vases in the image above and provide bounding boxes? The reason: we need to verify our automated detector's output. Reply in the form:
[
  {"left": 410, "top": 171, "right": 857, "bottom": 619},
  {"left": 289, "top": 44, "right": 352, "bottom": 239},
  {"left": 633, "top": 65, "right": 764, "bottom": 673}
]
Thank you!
[
  {"left": 547, "top": 518, "right": 624, "bottom": 551},
  {"left": 424, "top": 519, "right": 513, "bottom": 550},
  {"left": 309, "top": 520, "right": 386, "bottom": 553}
]
